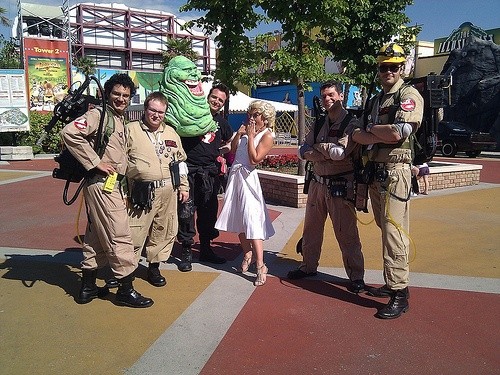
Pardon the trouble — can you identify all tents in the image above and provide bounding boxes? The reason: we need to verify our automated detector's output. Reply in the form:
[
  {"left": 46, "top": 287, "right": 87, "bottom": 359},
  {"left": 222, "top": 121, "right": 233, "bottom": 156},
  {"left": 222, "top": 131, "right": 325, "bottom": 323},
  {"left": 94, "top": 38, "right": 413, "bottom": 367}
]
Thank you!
[{"left": 199, "top": 78, "right": 299, "bottom": 148}]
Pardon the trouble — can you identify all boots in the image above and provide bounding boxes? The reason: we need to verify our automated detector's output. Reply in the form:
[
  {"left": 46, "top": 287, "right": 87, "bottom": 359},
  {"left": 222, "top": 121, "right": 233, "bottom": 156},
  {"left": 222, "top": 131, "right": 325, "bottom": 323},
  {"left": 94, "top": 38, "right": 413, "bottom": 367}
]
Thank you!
[
  {"left": 376, "top": 288, "right": 409, "bottom": 319},
  {"left": 105, "top": 273, "right": 135, "bottom": 287},
  {"left": 79, "top": 268, "right": 108, "bottom": 304},
  {"left": 368, "top": 283, "right": 410, "bottom": 299},
  {"left": 200, "top": 239, "right": 226, "bottom": 264},
  {"left": 179, "top": 243, "right": 193, "bottom": 271},
  {"left": 114, "top": 273, "right": 154, "bottom": 307},
  {"left": 147, "top": 262, "right": 167, "bottom": 287}
]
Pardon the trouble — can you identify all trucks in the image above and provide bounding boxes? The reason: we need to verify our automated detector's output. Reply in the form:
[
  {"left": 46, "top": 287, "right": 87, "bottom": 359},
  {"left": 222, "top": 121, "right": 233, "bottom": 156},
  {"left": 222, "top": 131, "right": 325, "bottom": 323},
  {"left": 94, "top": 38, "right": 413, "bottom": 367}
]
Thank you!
[{"left": 404, "top": 72, "right": 500, "bottom": 157}]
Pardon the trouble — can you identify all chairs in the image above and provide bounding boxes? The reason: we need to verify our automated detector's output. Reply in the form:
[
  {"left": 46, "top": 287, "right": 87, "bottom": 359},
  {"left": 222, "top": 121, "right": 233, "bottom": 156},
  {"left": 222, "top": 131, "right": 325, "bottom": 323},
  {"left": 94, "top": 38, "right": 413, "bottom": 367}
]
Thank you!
[{"left": 275, "top": 131, "right": 291, "bottom": 147}]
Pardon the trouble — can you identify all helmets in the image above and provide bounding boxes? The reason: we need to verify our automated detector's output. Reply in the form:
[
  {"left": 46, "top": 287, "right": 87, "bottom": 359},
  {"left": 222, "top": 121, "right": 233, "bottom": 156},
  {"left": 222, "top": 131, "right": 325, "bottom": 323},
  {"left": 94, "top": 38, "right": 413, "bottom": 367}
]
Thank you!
[{"left": 376, "top": 43, "right": 407, "bottom": 63}]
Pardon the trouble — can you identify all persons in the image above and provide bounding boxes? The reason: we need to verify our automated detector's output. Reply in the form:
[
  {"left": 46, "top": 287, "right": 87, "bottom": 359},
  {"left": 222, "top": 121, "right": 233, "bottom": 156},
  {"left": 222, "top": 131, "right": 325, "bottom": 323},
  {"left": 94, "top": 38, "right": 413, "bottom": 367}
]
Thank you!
[
  {"left": 287, "top": 81, "right": 368, "bottom": 293},
  {"left": 105, "top": 91, "right": 190, "bottom": 287},
  {"left": 215, "top": 101, "right": 276, "bottom": 287},
  {"left": 351, "top": 43, "right": 425, "bottom": 319},
  {"left": 62, "top": 74, "right": 155, "bottom": 309},
  {"left": 175, "top": 83, "right": 233, "bottom": 273},
  {"left": 410, "top": 161, "right": 430, "bottom": 197}
]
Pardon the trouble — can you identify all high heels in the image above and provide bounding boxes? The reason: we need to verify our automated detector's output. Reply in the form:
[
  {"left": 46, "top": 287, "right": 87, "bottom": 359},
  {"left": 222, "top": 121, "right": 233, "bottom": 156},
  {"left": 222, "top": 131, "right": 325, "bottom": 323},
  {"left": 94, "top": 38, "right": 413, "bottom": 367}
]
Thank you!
[
  {"left": 254, "top": 263, "right": 268, "bottom": 286},
  {"left": 237, "top": 249, "right": 257, "bottom": 272}
]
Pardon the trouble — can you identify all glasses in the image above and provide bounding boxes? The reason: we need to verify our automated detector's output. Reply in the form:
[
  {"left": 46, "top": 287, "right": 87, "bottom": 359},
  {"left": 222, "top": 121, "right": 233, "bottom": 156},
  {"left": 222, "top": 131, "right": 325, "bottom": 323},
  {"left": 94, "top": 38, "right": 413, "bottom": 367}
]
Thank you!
[{"left": 379, "top": 65, "right": 402, "bottom": 72}]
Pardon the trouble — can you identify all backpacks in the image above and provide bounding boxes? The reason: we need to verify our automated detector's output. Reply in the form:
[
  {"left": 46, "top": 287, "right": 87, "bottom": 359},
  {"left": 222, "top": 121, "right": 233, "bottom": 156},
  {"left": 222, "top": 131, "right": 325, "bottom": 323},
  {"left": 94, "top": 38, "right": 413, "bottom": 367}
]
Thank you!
[{"left": 366, "top": 82, "right": 437, "bottom": 165}]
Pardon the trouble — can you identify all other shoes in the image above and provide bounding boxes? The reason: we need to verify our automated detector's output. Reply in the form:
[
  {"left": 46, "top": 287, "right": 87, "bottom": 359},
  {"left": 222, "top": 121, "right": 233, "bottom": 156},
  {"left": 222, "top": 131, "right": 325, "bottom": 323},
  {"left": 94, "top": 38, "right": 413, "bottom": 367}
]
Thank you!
[
  {"left": 287, "top": 269, "right": 317, "bottom": 279},
  {"left": 352, "top": 279, "right": 365, "bottom": 293}
]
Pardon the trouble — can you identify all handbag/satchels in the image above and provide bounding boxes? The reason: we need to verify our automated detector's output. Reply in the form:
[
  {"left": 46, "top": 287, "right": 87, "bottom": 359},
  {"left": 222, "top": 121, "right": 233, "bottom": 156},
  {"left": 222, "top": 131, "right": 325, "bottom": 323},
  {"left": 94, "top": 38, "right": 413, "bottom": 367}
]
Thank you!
[{"left": 131, "top": 181, "right": 155, "bottom": 210}]
